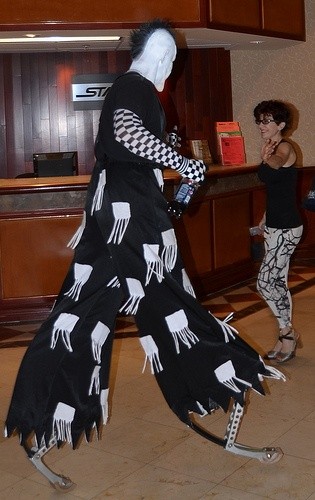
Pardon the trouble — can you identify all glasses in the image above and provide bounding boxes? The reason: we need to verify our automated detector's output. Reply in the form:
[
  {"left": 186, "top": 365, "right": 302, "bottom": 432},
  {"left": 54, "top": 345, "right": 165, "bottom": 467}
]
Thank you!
[{"left": 255, "top": 119, "right": 282, "bottom": 125}]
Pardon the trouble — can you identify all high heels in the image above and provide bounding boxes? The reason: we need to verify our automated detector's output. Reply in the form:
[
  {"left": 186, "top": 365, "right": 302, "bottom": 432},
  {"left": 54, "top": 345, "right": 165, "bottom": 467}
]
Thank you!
[{"left": 264, "top": 328, "right": 304, "bottom": 363}]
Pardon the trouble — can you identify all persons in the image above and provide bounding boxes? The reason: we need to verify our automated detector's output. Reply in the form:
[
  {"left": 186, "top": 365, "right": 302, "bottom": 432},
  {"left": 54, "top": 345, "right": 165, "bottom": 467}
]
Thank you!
[
  {"left": 8, "top": 19, "right": 263, "bottom": 380},
  {"left": 254, "top": 100, "right": 304, "bottom": 363}
]
